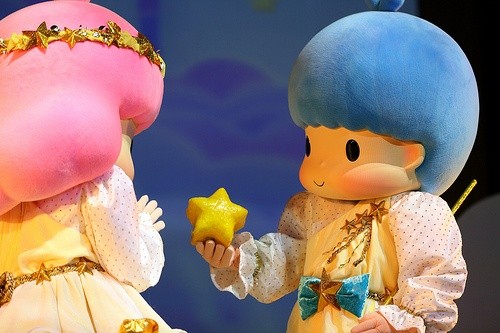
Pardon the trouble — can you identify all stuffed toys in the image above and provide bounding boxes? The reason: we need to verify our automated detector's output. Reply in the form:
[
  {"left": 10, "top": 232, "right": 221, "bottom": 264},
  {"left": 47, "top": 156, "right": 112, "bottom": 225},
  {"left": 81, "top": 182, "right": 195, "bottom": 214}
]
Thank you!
[
  {"left": 193, "top": 11, "right": 479, "bottom": 333},
  {"left": 0, "top": 1, "right": 189, "bottom": 331}
]
